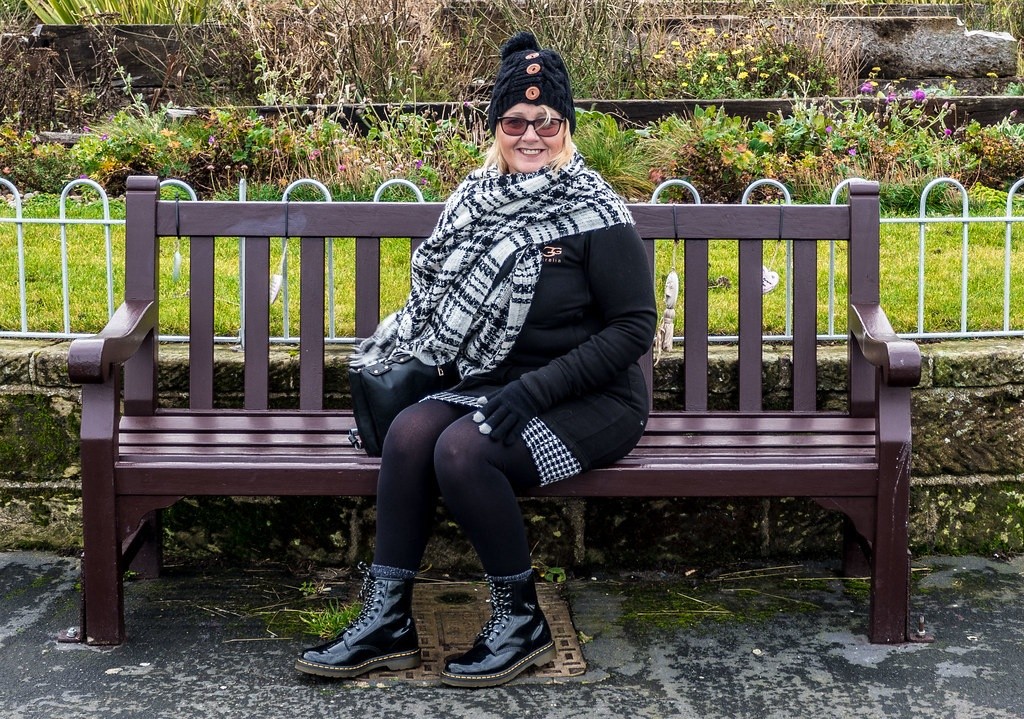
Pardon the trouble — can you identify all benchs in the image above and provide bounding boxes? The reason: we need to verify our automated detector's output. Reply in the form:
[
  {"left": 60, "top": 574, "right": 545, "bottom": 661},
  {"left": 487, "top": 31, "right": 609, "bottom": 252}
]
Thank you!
[{"left": 68, "top": 176, "right": 923, "bottom": 648}]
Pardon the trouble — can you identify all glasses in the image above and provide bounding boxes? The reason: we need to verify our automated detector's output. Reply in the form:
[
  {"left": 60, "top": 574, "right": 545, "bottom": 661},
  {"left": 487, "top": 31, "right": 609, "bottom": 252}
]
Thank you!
[{"left": 498, "top": 116, "right": 565, "bottom": 137}]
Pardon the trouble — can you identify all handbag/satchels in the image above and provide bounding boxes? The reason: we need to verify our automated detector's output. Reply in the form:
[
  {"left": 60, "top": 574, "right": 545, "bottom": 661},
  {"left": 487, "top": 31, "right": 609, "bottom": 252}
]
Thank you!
[{"left": 348, "top": 347, "right": 462, "bottom": 457}]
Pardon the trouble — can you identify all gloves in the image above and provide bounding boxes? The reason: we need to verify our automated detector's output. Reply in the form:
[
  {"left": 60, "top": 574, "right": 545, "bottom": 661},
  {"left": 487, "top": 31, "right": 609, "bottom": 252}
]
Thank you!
[{"left": 474, "top": 381, "right": 538, "bottom": 446}]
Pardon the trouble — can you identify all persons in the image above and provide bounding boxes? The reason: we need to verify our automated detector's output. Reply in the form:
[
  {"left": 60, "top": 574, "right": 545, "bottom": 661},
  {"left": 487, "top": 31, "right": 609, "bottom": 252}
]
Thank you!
[{"left": 292, "top": 47, "right": 661, "bottom": 691}]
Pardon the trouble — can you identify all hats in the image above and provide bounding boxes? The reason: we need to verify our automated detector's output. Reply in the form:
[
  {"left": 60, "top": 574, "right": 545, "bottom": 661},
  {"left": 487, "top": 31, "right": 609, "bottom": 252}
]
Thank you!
[{"left": 488, "top": 32, "right": 576, "bottom": 137}]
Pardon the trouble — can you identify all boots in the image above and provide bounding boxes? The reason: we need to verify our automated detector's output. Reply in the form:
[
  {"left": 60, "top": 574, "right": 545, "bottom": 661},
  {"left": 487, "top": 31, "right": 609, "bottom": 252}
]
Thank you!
[
  {"left": 296, "top": 561, "right": 422, "bottom": 679},
  {"left": 441, "top": 569, "right": 554, "bottom": 687}
]
